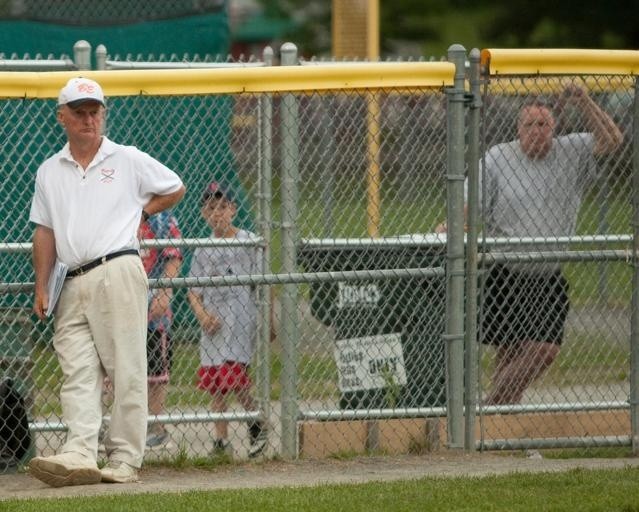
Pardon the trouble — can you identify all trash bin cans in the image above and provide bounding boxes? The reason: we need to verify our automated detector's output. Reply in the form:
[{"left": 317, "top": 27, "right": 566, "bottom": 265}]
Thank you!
[{"left": 295, "top": 246, "right": 449, "bottom": 410}]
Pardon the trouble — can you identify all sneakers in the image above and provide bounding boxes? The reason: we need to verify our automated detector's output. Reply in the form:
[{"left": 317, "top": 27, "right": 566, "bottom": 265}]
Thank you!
[
  {"left": 28, "top": 452, "right": 102, "bottom": 487},
  {"left": 195, "top": 439, "right": 234, "bottom": 467},
  {"left": 101, "top": 460, "right": 140, "bottom": 483},
  {"left": 146, "top": 429, "right": 173, "bottom": 451},
  {"left": 248, "top": 419, "right": 275, "bottom": 458}
]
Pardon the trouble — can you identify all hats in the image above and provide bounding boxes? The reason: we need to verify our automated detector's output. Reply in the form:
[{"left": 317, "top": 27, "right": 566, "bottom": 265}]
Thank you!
[
  {"left": 201, "top": 184, "right": 237, "bottom": 203},
  {"left": 58, "top": 78, "right": 106, "bottom": 109}
]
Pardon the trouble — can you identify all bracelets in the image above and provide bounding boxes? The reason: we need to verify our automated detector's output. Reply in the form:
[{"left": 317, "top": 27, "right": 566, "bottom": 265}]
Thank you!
[{"left": 142, "top": 211, "right": 150, "bottom": 221}]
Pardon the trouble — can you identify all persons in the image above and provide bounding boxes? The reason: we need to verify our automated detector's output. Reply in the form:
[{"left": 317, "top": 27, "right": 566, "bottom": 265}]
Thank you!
[
  {"left": 136, "top": 206, "right": 187, "bottom": 453},
  {"left": 26, "top": 75, "right": 188, "bottom": 487},
  {"left": 434, "top": 79, "right": 626, "bottom": 407},
  {"left": 185, "top": 182, "right": 279, "bottom": 467}
]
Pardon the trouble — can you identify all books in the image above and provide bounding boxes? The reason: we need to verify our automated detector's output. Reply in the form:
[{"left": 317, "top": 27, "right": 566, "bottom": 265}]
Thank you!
[{"left": 42, "top": 257, "right": 69, "bottom": 319}]
